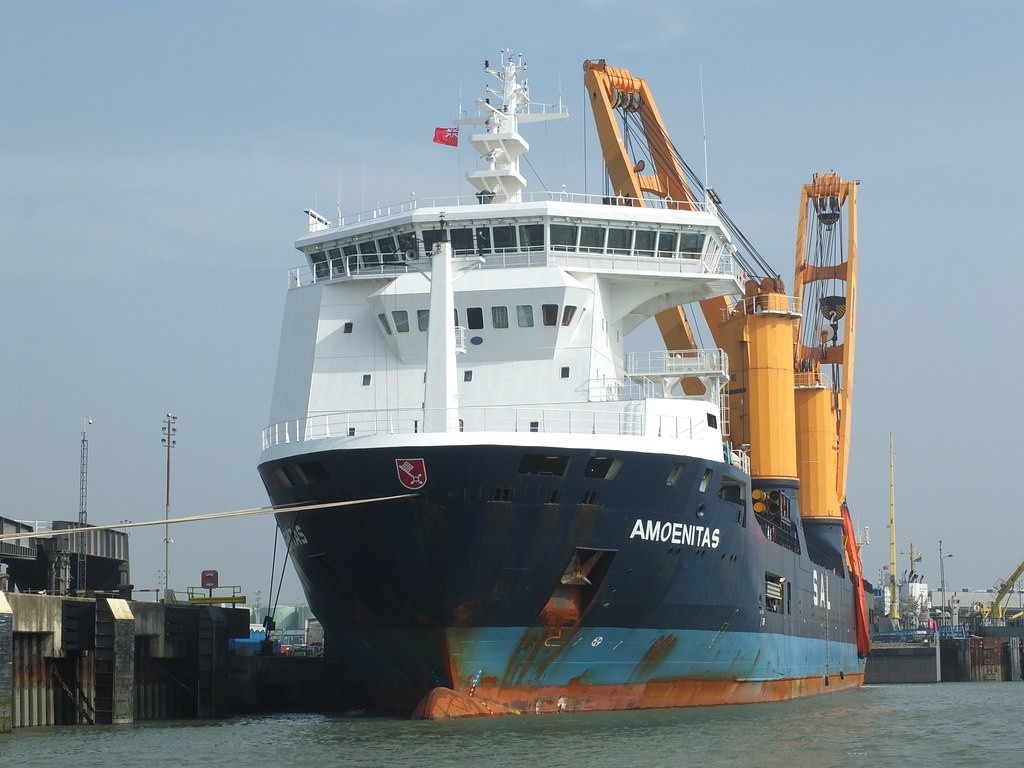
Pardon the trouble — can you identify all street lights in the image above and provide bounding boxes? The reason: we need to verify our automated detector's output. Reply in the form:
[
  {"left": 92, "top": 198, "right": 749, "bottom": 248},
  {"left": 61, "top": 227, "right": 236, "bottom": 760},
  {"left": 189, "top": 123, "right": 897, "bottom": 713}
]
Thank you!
[
  {"left": 161, "top": 411, "right": 178, "bottom": 591},
  {"left": 77, "top": 414, "right": 93, "bottom": 590},
  {"left": 942, "top": 554, "right": 953, "bottom": 626},
  {"left": 120, "top": 519, "right": 132, "bottom": 534}
]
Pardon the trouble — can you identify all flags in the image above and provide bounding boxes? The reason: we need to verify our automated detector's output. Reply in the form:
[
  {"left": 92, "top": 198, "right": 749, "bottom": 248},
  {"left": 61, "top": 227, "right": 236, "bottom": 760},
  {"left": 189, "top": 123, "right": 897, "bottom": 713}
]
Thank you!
[
  {"left": 882, "top": 564, "right": 889, "bottom": 571},
  {"left": 432, "top": 127, "right": 459, "bottom": 147}
]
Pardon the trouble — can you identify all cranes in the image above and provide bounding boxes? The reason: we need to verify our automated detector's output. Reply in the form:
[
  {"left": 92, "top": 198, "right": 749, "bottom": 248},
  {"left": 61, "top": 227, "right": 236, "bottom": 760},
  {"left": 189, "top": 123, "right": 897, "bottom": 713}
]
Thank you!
[
  {"left": 980, "top": 562, "right": 1024, "bottom": 624},
  {"left": 578, "top": 56, "right": 860, "bottom": 525}
]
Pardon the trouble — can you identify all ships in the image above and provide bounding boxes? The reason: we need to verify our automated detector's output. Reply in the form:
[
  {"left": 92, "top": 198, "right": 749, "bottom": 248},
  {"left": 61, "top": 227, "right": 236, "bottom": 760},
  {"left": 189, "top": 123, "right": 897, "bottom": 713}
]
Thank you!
[{"left": 252, "top": 48, "right": 871, "bottom": 720}]
